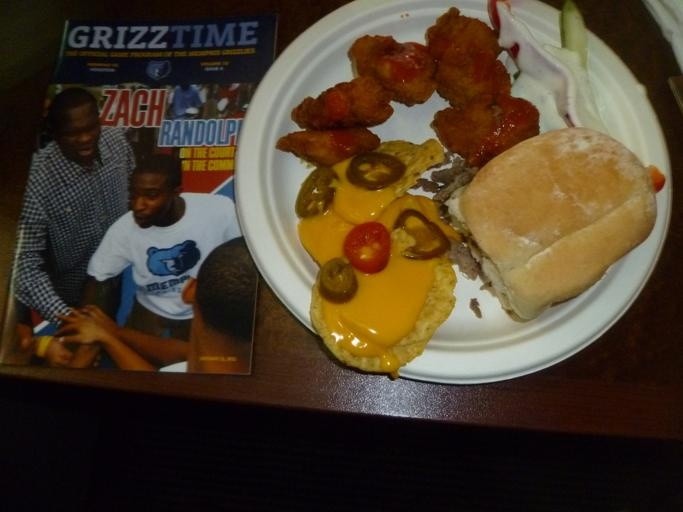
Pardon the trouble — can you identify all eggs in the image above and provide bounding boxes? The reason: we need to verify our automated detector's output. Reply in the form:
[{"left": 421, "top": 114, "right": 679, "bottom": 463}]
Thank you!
[{"left": 295, "top": 139, "right": 455, "bottom": 374}]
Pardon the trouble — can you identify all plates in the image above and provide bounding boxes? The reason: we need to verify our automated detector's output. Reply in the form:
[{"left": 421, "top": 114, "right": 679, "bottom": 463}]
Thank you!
[{"left": 234, "top": 0, "right": 675, "bottom": 385}]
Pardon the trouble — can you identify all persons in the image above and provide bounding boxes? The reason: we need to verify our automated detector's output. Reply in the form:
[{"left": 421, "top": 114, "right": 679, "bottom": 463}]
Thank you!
[{"left": 15, "top": 81, "right": 259, "bottom": 375}]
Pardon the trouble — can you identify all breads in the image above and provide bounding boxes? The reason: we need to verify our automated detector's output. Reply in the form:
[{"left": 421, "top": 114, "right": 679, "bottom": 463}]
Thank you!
[{"left": 446, "top": 127, "right": 658, "bottom": 323}]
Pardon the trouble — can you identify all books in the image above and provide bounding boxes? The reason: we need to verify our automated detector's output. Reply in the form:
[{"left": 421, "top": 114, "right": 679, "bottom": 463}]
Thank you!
[{"left": 0, "top": 16, "right": 279, "bottom": 376}]
